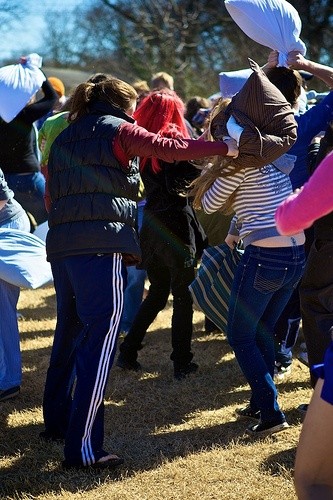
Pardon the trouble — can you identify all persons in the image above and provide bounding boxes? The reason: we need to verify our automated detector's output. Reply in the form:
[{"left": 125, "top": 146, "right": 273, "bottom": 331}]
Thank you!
[
  {"left": 43, "top": 79, "right": 239, "bottom": 470},
  {"left": 0, "top": 51, "right": 333, "bottom": 500}
]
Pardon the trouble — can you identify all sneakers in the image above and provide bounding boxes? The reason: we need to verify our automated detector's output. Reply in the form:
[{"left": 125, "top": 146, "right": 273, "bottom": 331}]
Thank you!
[
  {"left": 235, "top": 404, "right": 261, "bottom": 421},
  {"left": 246, "top": 422, "right": 290, "bottom": 437}
]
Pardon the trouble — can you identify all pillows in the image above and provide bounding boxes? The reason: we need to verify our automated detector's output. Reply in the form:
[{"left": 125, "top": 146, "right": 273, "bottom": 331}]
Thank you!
[
  {"left": 38, "top": 111, "right": 78, "bottom": 167},
  {"left": 0, "top": 53, "right": 47, "bottom": 123},
  {"left": 189, "top": 206, "right": 245, "bottom": 335},
  {"left": 212, "top": 69, "right": 298, "bottom": 167},
  {"left": 225, "top": 0, "right": 307, "bottom": 68},
  {"left": 0, "top": 228, "right": 54, "bottom": 290}
]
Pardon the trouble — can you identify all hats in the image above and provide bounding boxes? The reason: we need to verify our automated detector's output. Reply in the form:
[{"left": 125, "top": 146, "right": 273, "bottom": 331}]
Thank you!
[{"left": 47, "top": 76, "right": 65, "bottom": 96}]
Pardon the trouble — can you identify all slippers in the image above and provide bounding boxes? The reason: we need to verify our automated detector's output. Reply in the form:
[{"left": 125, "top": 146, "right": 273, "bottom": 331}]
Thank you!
[{"left": 90, "top": 454, "right": 124, "bottom": 469}]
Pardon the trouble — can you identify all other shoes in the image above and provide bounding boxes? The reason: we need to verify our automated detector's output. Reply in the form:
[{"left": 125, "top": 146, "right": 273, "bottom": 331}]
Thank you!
[
  {"left": 297, "top": 342, "right": 310, "bottom": 363},
  {"left": 273, "top": 360, "right": 291, "bottom": 380},
  {"left": 0, "top": 386, "right": 20, "bottom": 401},
  {"left": 173, "top": 359, "right": 198, "bottom": 381},
  {"left": 206, "top": 326, "right": 221, "bottom": 334},
  {"left": 297, "top": 404, "right": 309, "bottom": 414},
  {"left": 116, "top": 357, "right": 151, "bottom": 373}
]
